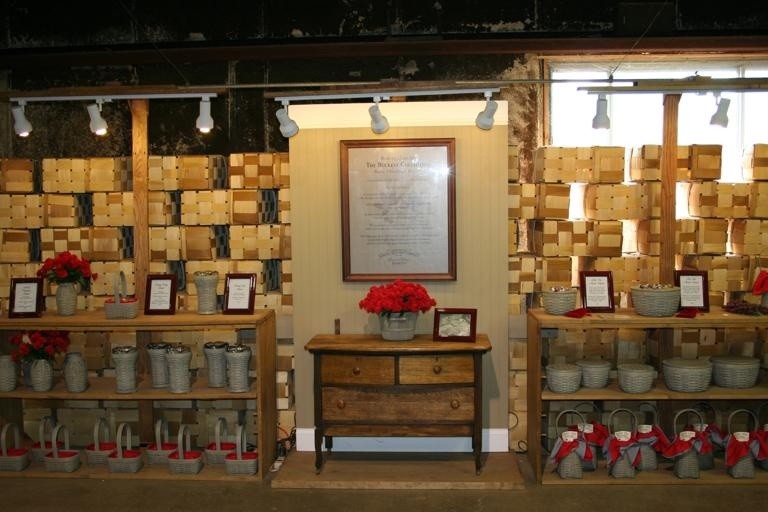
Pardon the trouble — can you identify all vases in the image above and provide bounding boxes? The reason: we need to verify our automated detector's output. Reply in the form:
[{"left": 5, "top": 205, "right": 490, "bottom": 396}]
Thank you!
[
  {"left": 53, "top": 276, "right": 78, "bottom": 315},
  {"left": 63, "top": 352, "right": 89, "bottom": 393},
  {"left": 632, "top": 280, "right": 681, "bottom": 319},
  {"left": 29, "top": 359, "right": 56, "bottom": 392},
  {"left": 540, "top": 281, "right": 581, "bottom": 318},
  {"left": 0, "top": 354, "right": 21, "bottom": 392},
  {"left": 542, "top": 355, "right": 764, "bottom": 397},
  {"left": 377, "top": 304, "right": 421, "bottom": 341},
  {"left": 193, "top": 272, "right": 219, "bottom": 315},
  {"left": 112, "top": 340, "right": 253, "bottom": 395}
]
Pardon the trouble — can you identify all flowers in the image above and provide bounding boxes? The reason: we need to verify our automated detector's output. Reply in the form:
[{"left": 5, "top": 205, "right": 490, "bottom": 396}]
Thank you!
[
  {"left": 357, "top": 277, "right": 437, "bottom": 316},
  {"left": 35, "top": 248, "right": 92, "bottom": 284},
  {"left": 8, "top": 329, "right": 71, "bottom": 363}
]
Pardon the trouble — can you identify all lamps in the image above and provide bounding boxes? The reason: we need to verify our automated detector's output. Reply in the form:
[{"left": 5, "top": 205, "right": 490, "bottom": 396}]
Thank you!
[{"left": 5, "top": 89, "right": 732, "bottom": 138}]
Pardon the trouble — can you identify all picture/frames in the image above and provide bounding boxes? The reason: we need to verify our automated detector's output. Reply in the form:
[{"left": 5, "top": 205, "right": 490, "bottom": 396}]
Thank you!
[
  {"left": 578, "top": 269, "right": 618, "bottom": 315},
  {"left": 672, "top": 266, "right": 712, "bottom": 313},
  {"left": 336, "top": 135, "right": 461, "bottom": 284},
  {"left": 9, "top": 275, "right": 44, "bottom": 320},
  {"left": 431, "top": 306, "right": 479, "bottom": 344},
  {"left": 144, "top": 273, "right": 179, "bottom": 317},
  {"left": 222, "top": 270, "right": 257, "bottom": 317}
]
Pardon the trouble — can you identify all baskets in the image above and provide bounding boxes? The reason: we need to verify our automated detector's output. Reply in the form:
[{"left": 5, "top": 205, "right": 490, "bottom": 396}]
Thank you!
[
  {"left": 543, "top": 289, "right": 576, "bottom": 314},
  {"left": 105, "top": 270, "right": 139, "bottom": 319},
  {"left": 631, "top": 286, "right": 680, "bottom": 316},
  {"left": 545, "top": 357, "right": 760, "bottom": 393},
  {"left": 1, "top": 416, "right": 258, "bottom": 475}
]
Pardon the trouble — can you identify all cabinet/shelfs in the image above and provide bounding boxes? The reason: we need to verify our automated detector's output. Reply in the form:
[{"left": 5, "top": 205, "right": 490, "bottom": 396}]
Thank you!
[
  {"left": 0, "top": 308, "right": 280, "bottom": 480},
  {"left": 521, "top": 307, "right": 767, "bottom": 486},
  {"left": 304, "top": 328, "right": 494, "bottom": 473}
]
